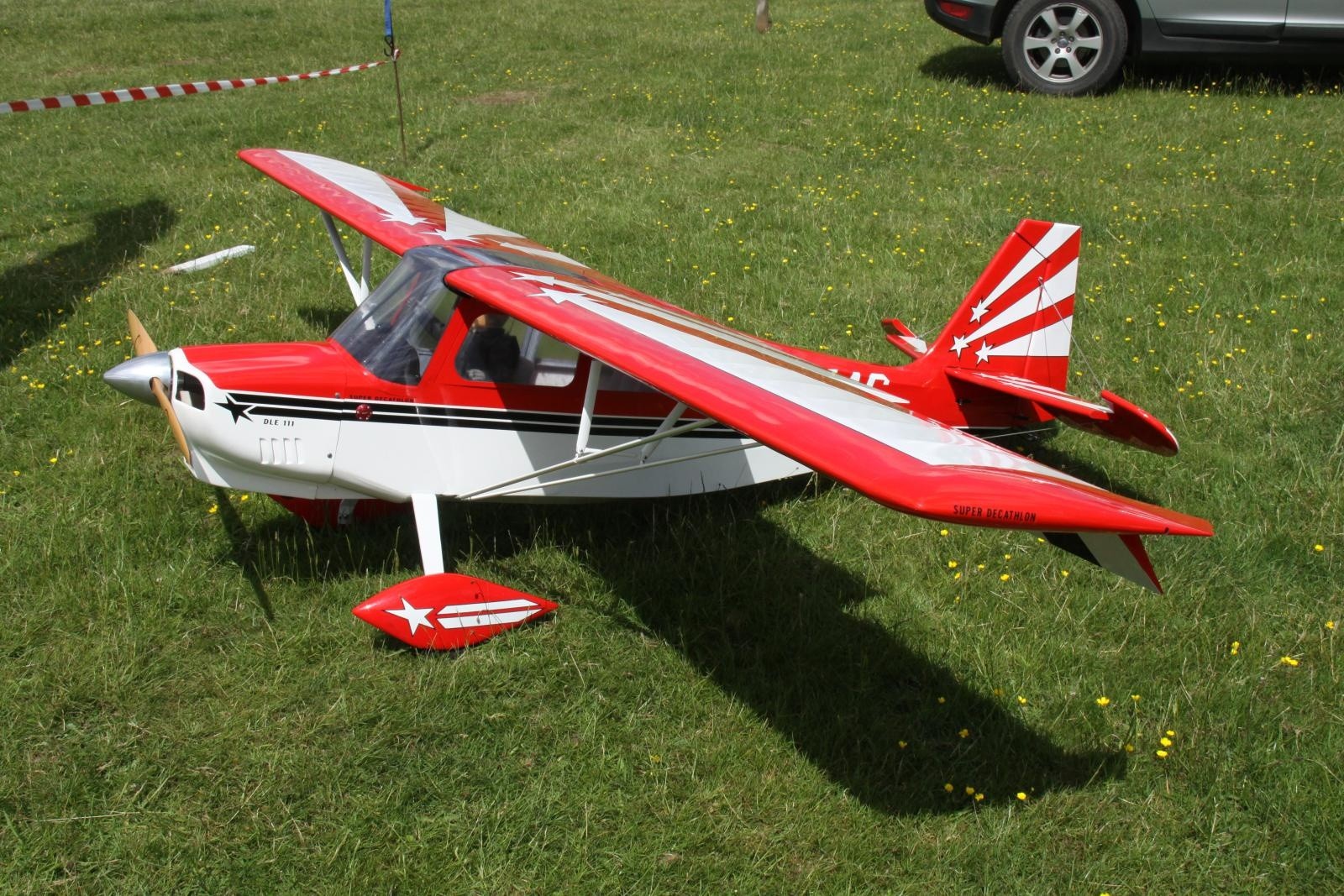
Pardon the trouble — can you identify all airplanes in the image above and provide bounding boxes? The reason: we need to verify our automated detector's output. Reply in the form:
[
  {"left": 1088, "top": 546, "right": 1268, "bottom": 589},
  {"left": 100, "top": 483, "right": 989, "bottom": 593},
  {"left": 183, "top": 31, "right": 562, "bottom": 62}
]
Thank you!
[{"left": 102, "top": 147, "right": 1215, "bottom": 648}]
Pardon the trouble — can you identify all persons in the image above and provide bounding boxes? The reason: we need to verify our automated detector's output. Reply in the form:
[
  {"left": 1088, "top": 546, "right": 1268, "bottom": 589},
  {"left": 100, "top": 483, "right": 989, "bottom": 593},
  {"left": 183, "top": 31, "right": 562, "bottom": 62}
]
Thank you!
[{"left": 408, "top": 301, "right": 520, "bottom": 381}]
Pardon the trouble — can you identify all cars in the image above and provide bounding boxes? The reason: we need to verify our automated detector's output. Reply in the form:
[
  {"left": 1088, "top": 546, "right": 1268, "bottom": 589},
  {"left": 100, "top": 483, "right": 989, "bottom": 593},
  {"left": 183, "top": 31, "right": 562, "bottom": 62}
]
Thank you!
[{"left": 924, "top": 0, "right": 1344, "bottom": 97}]
